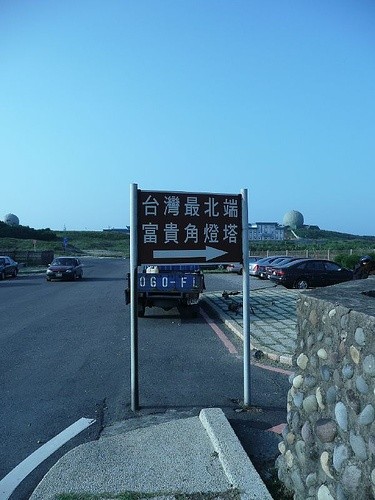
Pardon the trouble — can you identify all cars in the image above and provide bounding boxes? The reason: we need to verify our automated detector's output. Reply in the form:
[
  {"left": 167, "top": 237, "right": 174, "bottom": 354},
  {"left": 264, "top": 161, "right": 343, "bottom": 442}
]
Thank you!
[
  {"left": 0, "top": 256, "right": 19, "bottom": 280},
  {"left": 217, "top": 256, "right": 309, "bottom": 282},
  {"left": 268, "top": 259, "right": 355, "bottom": 289},
  {"left": 46, "top": 257, "right": 83, "bottom": 281}
]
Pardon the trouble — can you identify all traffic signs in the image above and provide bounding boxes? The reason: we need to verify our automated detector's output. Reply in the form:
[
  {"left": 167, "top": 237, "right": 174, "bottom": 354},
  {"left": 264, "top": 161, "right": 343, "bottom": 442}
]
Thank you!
[{"left": 136, "top": 189, "right": 245, "bottom": 266}]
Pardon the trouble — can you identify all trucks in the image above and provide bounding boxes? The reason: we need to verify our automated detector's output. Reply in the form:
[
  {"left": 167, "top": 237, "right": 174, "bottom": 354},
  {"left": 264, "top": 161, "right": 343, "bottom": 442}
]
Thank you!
[{"left": 124, "top": 266, "right": 206, "bottom": 317}]
